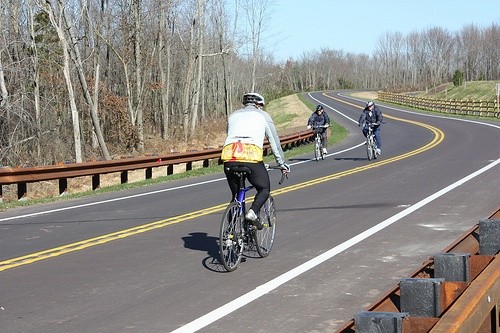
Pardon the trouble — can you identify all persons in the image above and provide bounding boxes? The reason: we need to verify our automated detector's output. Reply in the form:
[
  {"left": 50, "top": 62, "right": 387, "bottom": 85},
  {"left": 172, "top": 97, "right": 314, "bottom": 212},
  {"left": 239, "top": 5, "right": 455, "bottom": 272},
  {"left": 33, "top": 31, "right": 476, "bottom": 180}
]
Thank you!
[
  {"left": 358, "top": 101, "right": 383, "bottom": 155},
  {"left": 221, "top": 92, "right": 290, "bottom": 248},
  {"left": 307, "top": 105, "right": 330, "bottom": 154}
]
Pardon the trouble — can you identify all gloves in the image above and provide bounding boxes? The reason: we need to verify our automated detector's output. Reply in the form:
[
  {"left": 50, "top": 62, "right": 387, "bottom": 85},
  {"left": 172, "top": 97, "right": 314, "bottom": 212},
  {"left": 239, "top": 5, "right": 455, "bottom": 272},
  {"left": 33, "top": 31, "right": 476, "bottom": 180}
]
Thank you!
[
  {"left": 359, "top": 123, "right": 363, "bottom": 127},
  {"left": 377, "top": 121, "right": 380, "bottom": 124}
]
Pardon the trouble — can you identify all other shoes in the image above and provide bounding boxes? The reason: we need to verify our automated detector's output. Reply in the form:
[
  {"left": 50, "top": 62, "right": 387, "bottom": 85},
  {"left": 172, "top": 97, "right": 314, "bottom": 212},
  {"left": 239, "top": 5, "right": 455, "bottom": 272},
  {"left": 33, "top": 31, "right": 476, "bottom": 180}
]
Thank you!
[
  {"left": 376, "top": 149, "right": 381, "bottom": 154},
  {"left": 323, "top": 148, "right": 327, "bottom": 154}
]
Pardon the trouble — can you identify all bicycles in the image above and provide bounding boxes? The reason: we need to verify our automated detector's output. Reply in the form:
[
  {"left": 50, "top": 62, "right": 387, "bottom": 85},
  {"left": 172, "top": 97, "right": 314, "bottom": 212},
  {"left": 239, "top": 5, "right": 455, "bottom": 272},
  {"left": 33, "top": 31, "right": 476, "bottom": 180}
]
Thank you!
[
  {"left": 216, "top": 162, "right": 290, "bottom": 273},
  {"left": 361, "top": 121, "right": 379, "bottom": 161},
  {"left": 309, "top": 125, "right": 335, "bottom": 162}
]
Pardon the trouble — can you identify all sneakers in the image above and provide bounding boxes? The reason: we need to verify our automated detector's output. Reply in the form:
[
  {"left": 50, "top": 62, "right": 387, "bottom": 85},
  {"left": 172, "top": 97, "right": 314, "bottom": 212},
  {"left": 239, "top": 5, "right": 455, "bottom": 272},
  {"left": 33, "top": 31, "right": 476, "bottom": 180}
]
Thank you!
[{"left": 245, "top": 209, "right": 263, "bottom": 230}]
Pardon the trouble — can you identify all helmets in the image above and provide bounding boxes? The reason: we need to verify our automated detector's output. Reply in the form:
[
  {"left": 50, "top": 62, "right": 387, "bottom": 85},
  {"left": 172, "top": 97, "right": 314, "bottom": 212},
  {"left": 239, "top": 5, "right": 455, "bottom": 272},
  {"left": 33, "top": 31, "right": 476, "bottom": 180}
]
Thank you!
[
  {"left": 316, "top": 105, "right": 323, "bottom": 111},
  {"left": 366, "top": 101, "right": 374, "bottom": 107},
  {"left": 243, "top": 93, "right": 264, "bottom": 106}
]
